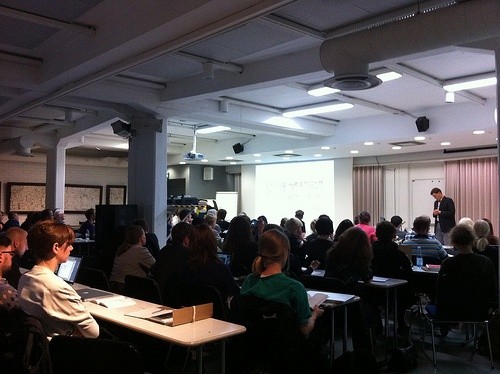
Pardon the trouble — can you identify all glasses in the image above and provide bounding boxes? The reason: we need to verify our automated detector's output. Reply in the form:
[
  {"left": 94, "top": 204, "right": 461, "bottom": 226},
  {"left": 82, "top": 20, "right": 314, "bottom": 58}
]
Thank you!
[{"left": 1, "top": 251, "right": 15, "bottom": 256}]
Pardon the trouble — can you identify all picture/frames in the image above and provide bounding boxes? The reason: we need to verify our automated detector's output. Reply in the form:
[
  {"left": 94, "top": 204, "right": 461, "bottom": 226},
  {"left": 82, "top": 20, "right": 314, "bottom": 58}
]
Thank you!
[
  {"left": 7, "top": 182, "right": 103, "bottom": 214},
  {"left": 106, "top": 185, "right": 127, "bottom": 205}
]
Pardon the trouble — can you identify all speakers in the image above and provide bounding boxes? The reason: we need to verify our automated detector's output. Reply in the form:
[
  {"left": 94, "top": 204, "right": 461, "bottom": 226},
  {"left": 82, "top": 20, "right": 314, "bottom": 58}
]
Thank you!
[
  {"left": 414, "top": 116, "right": 429, "bottom": 132},
  {"left": 94, "top": 204, "right": 137, "bottom": 278},
  {"left": 233, "top": 143, "right": 244, "bottom": 154},
  {"left": 110, "top": 120, "right": 132, "bottom": 138}
]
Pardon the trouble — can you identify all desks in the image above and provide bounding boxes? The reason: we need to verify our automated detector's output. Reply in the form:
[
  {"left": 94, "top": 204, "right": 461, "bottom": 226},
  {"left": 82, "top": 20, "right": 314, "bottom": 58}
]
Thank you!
[
  {"left": 306, "top": 287, "right": 360, "bottom": 367},
  {"left": 71, "top": 283, "right": 246, "bottom": 374},
  {"left": 301, "top": 267, "right": 408, "bottom": 374},
  {"left": 412, "top": 265, "right": 438, "bottom": 274}
]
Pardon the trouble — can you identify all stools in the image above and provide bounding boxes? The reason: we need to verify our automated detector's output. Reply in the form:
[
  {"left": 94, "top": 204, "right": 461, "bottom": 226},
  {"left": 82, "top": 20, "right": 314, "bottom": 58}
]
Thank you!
[{"left": 425, "top": 314, "right": 494, "bottom": 370}]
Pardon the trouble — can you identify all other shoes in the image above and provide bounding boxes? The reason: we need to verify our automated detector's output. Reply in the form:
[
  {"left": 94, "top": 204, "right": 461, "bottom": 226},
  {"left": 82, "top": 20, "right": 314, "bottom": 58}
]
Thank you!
[
  {"left": 439, "top": 327, "right": 448, "bottom": 337},
  {"left": 398, "top": 323, "right": 410, "bottom": 333}
]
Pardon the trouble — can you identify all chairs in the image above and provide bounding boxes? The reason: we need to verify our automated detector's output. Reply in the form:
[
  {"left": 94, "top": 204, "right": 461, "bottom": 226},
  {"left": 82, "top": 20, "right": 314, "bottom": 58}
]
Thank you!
[
  {"left": 228, "top": 293, "right": 328, "bottom": 374},
  {"left": 164, "top": 280, "right": 226, "bottom": 373},
  {"left": 48, "top": 336, "right": 144, "bottom": 374},
  {"left": 126, "top": 275, "right": 158, "bottom": 300},
  {"left": 78, "top": 267, "right": 110, "bottom": 291}
]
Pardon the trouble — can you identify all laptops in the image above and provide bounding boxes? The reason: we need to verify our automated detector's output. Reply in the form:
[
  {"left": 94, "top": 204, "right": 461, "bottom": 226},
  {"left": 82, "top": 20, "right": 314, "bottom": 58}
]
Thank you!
[{"left": 55, "top": 255, "right": 81, "bottom": 284}]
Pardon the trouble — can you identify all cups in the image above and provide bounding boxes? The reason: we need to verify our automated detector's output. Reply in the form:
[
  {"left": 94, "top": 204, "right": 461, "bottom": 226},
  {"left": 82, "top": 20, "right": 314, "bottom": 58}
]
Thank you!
[{"left": 405, "top": 235, "right": 410, "bottom": 240}]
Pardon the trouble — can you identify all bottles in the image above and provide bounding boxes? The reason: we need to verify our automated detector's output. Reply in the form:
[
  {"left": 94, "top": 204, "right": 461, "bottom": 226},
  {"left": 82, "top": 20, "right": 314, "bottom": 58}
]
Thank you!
[
  {"left": 85, "top": 227, "right": 90, "bottom": 240},
  {"left": 416, "top": 246, "right": 423, "bottom": 267}
]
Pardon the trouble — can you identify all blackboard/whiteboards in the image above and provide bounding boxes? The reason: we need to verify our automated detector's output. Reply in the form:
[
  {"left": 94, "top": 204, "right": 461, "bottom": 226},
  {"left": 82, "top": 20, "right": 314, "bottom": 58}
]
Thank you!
[{"left": 411, "top": 178, "right": 445, "bottom": 225}]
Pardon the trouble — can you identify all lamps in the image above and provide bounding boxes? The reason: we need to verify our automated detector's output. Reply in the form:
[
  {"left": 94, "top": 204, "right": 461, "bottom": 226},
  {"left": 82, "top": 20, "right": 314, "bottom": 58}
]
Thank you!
[
  {"left": 307, "top": 68, "right": 402, "bottom": 96},
  {"left": 443, "top": 71, "right": 497, "bottom": 92},
  {"left": 282, "top": 100, "right": 355, "bottom": 119}
]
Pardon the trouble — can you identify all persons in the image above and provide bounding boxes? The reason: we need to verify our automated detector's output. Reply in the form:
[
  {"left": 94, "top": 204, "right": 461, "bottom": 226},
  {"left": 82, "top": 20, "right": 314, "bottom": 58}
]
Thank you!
[
  {"left": 0, "top": 208, "right": 100, "bottom": 340},
  {"left": 431, "top": 187, "right": 456, "bottom": 245},
  {"left": 110, "top": 208, "right": 500, "bottom": 374}
]
